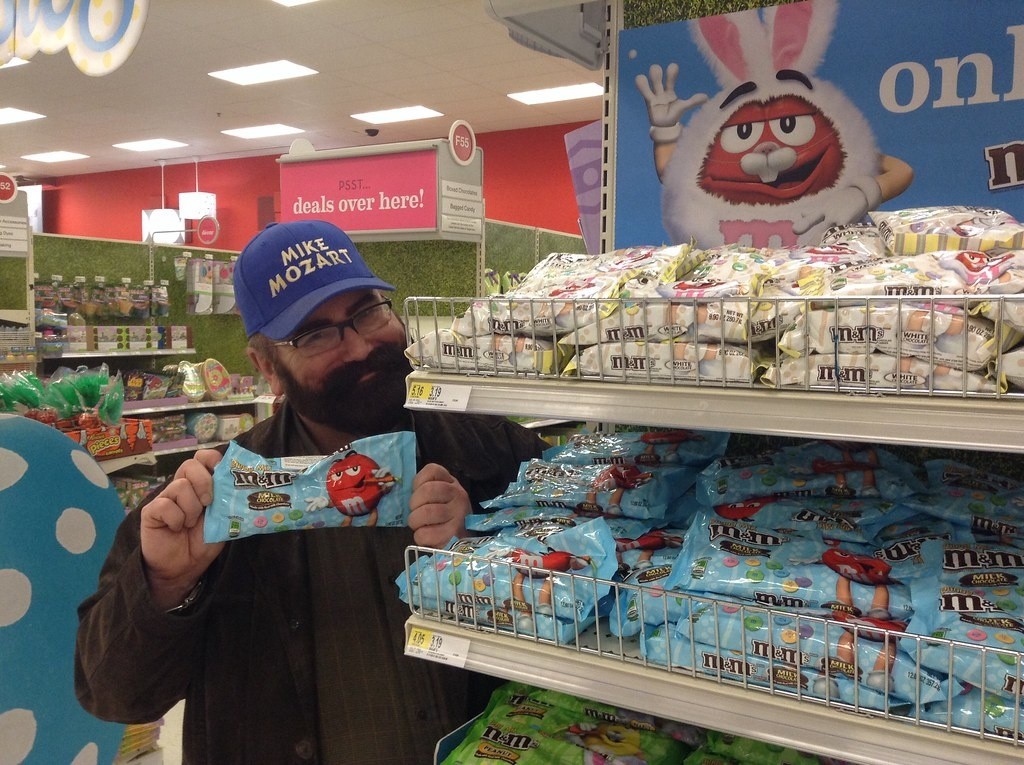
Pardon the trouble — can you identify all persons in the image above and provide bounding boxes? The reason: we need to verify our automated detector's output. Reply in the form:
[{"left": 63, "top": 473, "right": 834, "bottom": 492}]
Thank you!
[{"left": 74, "top": 218, "right": 554, "bottom": 765}]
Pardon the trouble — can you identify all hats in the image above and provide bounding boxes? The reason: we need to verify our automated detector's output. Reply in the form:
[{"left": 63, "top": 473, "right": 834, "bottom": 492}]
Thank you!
[{"left": 231, "top": 221, "right": 394, "bottom": 340}]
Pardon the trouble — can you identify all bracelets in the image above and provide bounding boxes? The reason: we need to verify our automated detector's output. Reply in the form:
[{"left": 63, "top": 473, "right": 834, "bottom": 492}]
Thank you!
[{"left": 166, "top": 580, "right": 201, "bottom": 614}]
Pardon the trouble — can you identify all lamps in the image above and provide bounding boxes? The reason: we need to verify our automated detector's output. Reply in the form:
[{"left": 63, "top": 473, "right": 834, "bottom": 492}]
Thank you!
[
  {"left": 141, "top": 158, "right": 192, "bottom": 246},
  {"left": 178, "top": 156, "right": 216, "bottom": 220}
]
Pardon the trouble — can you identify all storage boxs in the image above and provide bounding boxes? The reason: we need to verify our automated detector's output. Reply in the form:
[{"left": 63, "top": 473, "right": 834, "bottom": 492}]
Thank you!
[{"left": 3, "top": 323, "right": 198, "bottom": 465}]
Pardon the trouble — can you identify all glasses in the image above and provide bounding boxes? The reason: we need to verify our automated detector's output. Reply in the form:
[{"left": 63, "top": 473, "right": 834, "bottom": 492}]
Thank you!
[{"left": 266, "top": 292, "right": 393, "bottom": 357}]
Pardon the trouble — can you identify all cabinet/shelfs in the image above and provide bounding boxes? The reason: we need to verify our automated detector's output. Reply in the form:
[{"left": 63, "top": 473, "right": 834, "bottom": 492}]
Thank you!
[
  {"left": 404, "top": 294, "right": 1024, "bottom": 765},
  {"left": 45, "top": 349, "right": 276, "bottom": 455},
  {"left": 0, "top": 307, "right": 158, "bottom": 483}
]
